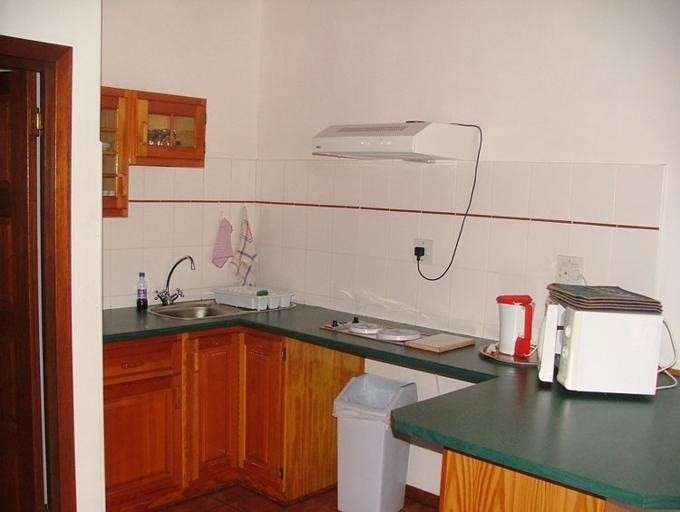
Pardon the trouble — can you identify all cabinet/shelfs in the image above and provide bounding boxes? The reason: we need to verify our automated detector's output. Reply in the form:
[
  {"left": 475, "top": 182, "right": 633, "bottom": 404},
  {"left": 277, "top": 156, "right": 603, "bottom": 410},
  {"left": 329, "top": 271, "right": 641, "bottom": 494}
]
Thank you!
[{"left": 136, "top": 272, "right": 148, "bottom": 310}]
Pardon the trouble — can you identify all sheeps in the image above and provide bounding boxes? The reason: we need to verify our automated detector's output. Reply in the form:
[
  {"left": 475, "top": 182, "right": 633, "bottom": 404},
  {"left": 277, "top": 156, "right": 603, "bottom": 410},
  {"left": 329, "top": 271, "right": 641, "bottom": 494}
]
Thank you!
[{"left": 154, "top": 255, "right": 196, "bottom": 307}]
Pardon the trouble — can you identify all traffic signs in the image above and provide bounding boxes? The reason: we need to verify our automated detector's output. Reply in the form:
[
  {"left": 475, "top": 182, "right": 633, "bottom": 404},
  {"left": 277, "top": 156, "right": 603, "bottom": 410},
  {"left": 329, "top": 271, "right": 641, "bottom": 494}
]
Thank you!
[
  {"left": 554, "top": 254, "right": 585, "bottom": 284},
  {"left": 412, "top": 238, "right": 432, "bottom": 265}
]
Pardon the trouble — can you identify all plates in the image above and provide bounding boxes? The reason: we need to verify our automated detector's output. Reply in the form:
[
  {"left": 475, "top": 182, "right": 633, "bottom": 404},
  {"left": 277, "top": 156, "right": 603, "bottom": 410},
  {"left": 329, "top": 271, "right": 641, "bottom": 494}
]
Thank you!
[
  {"left": 101, "top": 87, "right": 129, "bottom": 216},
  {"left": 103, "top": 333, "right": 194, "bottom": 512},
  {"left": 238, "top": 324, "right": 365, "bottom": 507},
  {"left": 128, "top": 89, "right": 207, "bottom": 168},
  {"left": 438, "top": 448, "right": 640, "bottom": 512},
  {"left": 189, "top": 326, "right": 238, "bottom": 498}
]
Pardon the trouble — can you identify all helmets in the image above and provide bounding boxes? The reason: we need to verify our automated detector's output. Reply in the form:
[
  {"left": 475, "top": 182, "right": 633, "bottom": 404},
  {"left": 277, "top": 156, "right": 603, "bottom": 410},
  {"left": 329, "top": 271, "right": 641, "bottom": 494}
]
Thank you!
[{"left": 480, "top": 343, "right": 538, "bottom": 368}]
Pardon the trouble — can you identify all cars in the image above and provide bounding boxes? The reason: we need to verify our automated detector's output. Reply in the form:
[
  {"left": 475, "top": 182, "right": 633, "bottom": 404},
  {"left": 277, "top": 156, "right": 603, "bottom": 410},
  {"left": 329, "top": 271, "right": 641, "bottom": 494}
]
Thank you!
[
  {"left": 497, "top": 295, "right": 534, "bottom": 357},
  {"left": 148, "top": 128, "right": 177, "bottom": 146}
]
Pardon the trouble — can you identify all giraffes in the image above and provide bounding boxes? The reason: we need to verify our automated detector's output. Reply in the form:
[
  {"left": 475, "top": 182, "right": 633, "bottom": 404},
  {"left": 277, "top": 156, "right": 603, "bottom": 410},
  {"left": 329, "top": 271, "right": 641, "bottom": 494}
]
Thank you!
[{"left": 319, "top": 317, "right": 429, "bottom": 349}]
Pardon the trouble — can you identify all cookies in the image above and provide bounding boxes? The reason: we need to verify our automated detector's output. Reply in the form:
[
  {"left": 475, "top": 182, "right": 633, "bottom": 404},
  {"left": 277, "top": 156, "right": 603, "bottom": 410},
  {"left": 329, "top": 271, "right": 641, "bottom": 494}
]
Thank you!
[{"left": 536, "top": 298, "right": 661, "bottom": 399}]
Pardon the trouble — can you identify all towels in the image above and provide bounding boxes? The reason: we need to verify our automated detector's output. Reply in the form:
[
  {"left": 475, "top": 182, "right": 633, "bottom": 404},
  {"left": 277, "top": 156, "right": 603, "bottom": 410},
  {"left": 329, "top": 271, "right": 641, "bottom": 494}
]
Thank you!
[{"left": 331, "top": 373, "right": 419, "bottom": 512}]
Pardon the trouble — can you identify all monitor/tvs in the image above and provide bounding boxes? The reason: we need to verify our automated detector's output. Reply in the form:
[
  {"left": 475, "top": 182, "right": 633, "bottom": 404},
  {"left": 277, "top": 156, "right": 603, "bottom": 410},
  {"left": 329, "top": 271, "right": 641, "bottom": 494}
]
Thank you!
[{"left": 407, "top": 333, "right": 473, "bottom": 353}]
